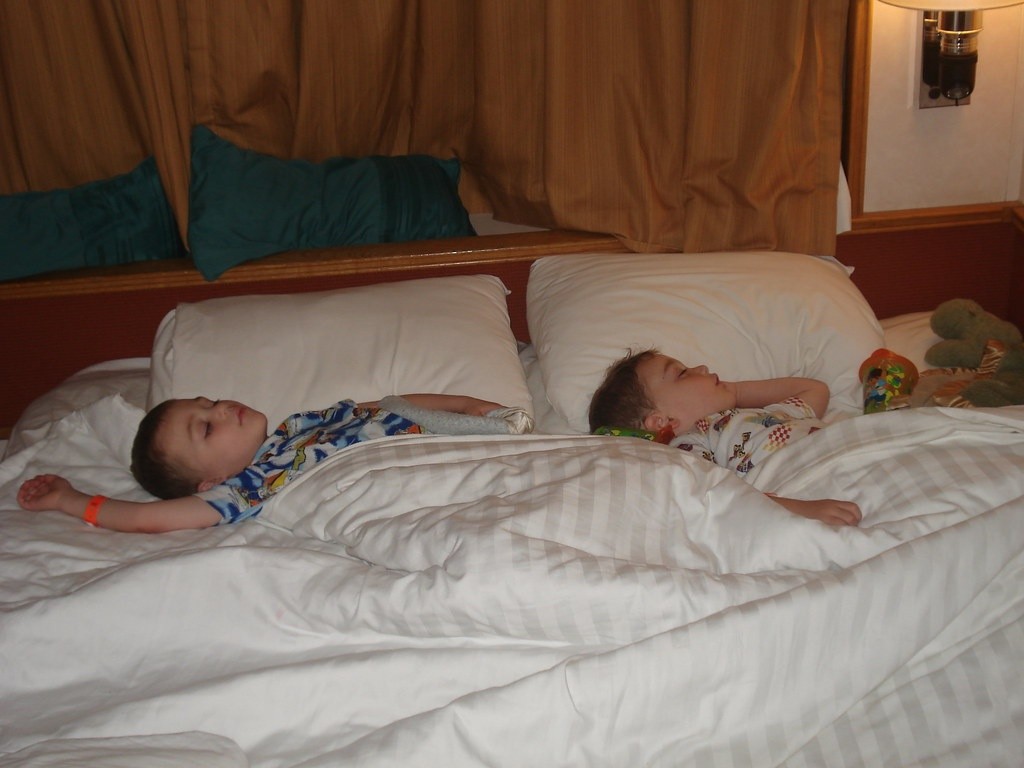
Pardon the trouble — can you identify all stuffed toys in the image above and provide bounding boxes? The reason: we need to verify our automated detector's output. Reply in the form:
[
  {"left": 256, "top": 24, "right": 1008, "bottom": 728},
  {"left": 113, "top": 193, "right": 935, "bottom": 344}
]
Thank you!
[{"left": 924, "top": 298, "right": 1024, "bottom": 407}]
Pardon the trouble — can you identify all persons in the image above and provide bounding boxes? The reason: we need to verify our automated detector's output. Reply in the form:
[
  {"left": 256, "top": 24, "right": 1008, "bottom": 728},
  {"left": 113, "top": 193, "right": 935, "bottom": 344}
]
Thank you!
[
  {"left": 588, "top": 345, "right": 863, "bottom": 525},
  {"left": 17, "top": 393, "right": 509, "bottom": 534}
]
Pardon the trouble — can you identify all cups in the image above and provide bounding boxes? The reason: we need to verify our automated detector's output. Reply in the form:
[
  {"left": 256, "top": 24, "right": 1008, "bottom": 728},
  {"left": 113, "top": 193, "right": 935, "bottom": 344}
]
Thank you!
[{"left": 859, "top": 349, "right": 918, "bottom": 414}]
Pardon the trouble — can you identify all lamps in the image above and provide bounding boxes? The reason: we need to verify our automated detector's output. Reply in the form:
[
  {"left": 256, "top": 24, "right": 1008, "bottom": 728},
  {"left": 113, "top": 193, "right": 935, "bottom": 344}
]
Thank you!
[{"left": 879, "top": 0, "right": 1024, "bottom": 110}]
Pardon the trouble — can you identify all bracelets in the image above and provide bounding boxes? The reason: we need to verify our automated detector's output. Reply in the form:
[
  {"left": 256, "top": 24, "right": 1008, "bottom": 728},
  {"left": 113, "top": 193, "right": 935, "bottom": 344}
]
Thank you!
[{"left": 82, "top": 495, "right": 109, "bottom": 527}]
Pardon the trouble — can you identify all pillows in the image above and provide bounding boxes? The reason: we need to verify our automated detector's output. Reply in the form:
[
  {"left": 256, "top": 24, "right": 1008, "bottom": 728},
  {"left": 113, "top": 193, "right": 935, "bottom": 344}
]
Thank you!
[
  {"left": 187, "top": 125, "right": 477, "bottom": 282},
  {"left": 1, "top": 155, "right": 182, "bottom": 282},
  {"left": 524, "top": 251, "right": 890, "bottom": 432},
  {"left": 147, "top": 275, "right": 534, "bottom": 420}
]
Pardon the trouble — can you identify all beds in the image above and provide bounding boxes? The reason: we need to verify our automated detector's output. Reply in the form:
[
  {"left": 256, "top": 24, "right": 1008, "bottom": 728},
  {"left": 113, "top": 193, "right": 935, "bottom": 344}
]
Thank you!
[{"left": 0, "top": 199, "right": 1023, "bottom": 768}]
